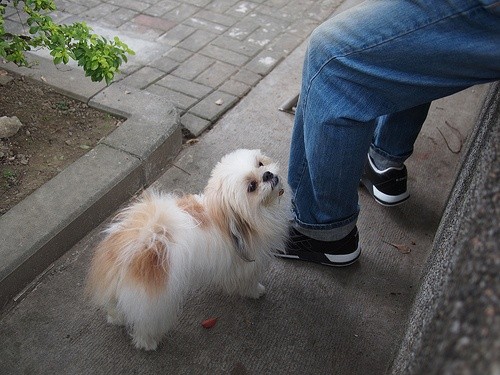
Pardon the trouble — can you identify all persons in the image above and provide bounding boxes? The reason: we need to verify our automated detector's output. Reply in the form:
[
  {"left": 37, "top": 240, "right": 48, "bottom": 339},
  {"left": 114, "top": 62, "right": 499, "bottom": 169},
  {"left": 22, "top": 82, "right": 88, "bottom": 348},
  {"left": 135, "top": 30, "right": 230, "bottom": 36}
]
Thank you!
[{"left": 274, "top": 0, "right": 500, "bottom": 269}]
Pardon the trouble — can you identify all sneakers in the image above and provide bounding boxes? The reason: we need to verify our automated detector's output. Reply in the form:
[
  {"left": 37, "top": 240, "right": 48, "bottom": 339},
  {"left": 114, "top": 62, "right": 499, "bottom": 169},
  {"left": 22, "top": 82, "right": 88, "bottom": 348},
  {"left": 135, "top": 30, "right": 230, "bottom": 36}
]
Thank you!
[
  {"left": 270, "top": 225, "right": 362, "bottom": 266},
  {"left": 360, "top": 153, "right": 410, "bottom": 207}
]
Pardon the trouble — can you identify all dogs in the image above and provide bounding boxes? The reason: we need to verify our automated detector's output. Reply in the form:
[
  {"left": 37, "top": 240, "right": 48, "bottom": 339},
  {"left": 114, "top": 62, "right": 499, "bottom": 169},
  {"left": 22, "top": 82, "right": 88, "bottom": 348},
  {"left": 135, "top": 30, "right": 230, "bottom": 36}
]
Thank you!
[{"left": 86, "top": 148, "right": 296, "bottom": 352}]
